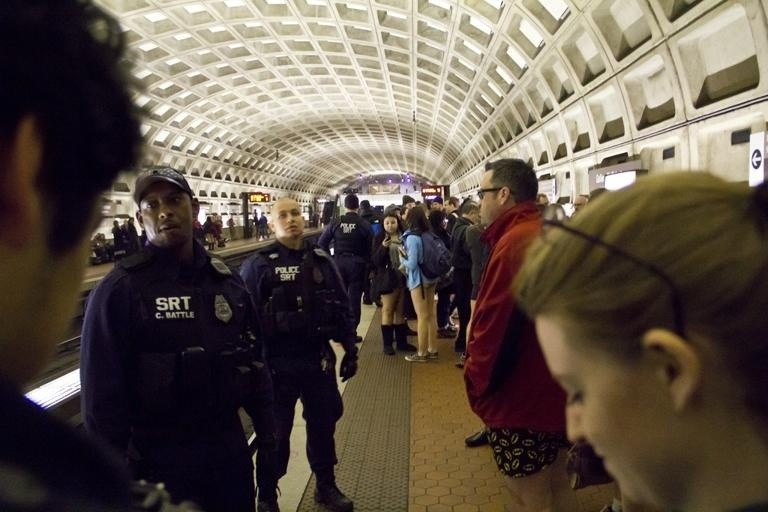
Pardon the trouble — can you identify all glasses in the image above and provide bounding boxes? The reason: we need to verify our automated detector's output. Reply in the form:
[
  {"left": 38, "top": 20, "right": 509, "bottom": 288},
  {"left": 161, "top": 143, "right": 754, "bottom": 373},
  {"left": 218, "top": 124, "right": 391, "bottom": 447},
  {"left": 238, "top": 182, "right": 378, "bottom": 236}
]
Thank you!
[
  {"left": 534, "top": 197, "right": 693, "bottom": 339},
  {"left": 477, "top": 183, "right": 514, "bottom": 200}
]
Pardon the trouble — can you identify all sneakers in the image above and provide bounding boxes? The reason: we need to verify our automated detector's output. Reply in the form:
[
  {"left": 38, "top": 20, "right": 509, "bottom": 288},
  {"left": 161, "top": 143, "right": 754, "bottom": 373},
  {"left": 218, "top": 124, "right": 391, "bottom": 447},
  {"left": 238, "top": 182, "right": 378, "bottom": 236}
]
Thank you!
[
  {"left": 404, "top": 351, "right": 429, "bottom": 363},
  {"left": 454, "top": 349, "right": 467, "bottom": 369},
  {"left": 407, "top": 325, "right": 419, "bottom": 336},
  {"left": 427, "top": 349, "right": 440, "bottom": 360},
  {"left": 436, "top": 316, "right": 458, "bottom": 338}
]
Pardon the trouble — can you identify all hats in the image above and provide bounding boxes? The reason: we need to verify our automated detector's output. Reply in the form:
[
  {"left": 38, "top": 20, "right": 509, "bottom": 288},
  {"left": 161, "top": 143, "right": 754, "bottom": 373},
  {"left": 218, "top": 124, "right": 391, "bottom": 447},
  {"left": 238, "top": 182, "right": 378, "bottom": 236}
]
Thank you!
[
  {"left": 432, "top": 196, "right": 444, "bottom": 205},
  {"left": 131, "top": 164, "right": 195, "bottom": 205},
  {"left": 402, "top": 195, "right": 416, "bottom": 208}
]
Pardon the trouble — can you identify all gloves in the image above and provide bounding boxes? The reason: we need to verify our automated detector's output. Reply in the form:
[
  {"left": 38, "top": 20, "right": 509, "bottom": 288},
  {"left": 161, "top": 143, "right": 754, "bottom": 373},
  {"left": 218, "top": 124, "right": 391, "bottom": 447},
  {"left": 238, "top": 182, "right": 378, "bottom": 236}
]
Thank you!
[{"left": 340, "top": 355, "right": 359, "bottom": 383}]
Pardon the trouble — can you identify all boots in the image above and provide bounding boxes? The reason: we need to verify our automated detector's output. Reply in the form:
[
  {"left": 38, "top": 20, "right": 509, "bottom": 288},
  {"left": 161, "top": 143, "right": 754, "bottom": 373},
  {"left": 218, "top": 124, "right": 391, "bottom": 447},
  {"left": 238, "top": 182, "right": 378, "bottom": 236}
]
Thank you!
[
  {"left": 256, "top": 483, "right": 283, "bottom": 512},
  {"left": 309, "top": 464, "right": 355, "bottom": 512},
  {"left": 380, "top": 324, "right": 398, "bottom": 356},
  {"left": 393, "top": 322, "right": 418, "bottom": 353}
]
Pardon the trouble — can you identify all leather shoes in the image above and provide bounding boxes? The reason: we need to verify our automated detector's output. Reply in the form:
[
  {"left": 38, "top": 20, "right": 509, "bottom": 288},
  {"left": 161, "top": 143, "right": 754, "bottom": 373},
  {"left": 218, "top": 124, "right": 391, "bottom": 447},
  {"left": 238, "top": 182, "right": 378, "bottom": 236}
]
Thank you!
[{"left": 464, "top": 429, "right": 490, "bottom": 448}]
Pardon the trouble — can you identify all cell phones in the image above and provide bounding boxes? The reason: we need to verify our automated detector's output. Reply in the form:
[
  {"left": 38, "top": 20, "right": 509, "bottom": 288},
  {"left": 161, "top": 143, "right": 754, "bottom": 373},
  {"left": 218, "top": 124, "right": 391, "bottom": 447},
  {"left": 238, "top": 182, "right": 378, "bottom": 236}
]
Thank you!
[{"left": 386, "top": 236, "right": 391, "bottom": 242}]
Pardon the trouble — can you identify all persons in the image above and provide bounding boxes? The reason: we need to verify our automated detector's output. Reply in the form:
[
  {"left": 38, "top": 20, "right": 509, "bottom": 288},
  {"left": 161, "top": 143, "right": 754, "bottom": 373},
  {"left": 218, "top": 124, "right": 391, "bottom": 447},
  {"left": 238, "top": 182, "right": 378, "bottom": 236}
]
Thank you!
[
  {"left": 90, "top": 207, "right": 272, "bottom": 264},
  {"left": 240, "top": 196, "right": 361, "bottom": 511},
  {"left": 79, "top": 164, "right": 281, "bottom": 511},
  {"left": 316, "top": 195, "right": 486, "bottom": 363},
  {"left": 0, "top": 1, "right": 159, "bottom": 512},
  {"left": 461, "top": 159, "right": 768, "bottom": 512}
]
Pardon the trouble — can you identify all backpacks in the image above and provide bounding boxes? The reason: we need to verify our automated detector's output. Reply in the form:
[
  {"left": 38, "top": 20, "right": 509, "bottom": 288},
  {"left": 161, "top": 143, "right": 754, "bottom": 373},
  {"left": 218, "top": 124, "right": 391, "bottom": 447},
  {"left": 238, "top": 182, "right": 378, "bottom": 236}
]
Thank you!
[{"left": 403, "top": 228, "right": 453, "bottom": 280}]
